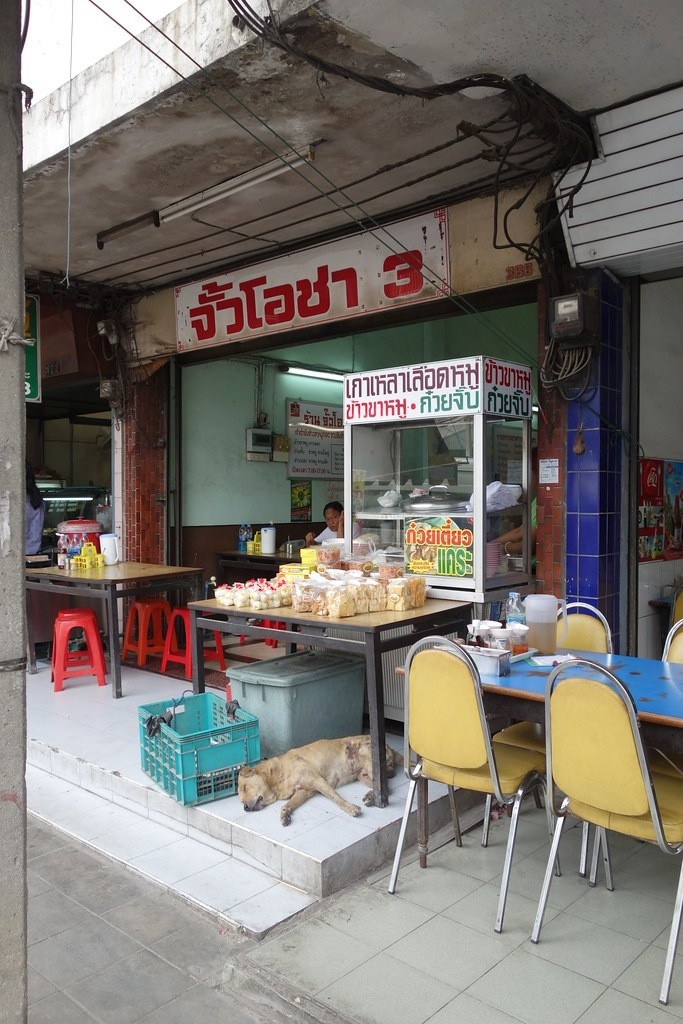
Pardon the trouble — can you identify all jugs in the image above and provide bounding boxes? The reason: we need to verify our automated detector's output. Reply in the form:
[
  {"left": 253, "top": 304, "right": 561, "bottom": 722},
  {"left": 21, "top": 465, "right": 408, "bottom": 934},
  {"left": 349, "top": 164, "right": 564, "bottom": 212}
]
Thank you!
[
  {"left": 99, "top": 533, "right": 120, "bottom": 566},
  {"left": 520, "top": 594, "right": 568, "bottom": 655},
  {"left": 262, "top": 527, "right": 276, "bottom": 554}
]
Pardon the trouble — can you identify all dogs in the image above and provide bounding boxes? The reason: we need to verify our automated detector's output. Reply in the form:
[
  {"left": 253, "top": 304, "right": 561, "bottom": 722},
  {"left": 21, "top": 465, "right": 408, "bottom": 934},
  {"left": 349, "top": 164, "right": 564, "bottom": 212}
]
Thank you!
[{"left": 237, "top": 734, "right": 417, "bottom": 826}]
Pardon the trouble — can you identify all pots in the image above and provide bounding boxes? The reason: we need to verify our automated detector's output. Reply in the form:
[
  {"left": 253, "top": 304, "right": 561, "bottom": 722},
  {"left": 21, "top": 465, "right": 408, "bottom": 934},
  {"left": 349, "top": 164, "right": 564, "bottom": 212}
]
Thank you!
[{"left": 55, "top": 516, "right": 105, "bottom": 554}]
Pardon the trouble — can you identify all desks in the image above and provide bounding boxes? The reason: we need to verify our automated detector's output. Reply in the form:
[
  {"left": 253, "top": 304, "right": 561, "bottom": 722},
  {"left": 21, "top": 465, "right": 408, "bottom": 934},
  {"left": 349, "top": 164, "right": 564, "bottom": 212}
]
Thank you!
[
  {"left": 216, "top": 548, "right": 302, "bottom": 636},
  {"left": 187, "top": 597, "right": 475, "bottom": 808},
  {"left": 394, "top": 648, "right": 683, "bottom": 869},
  {"left": 24, "top": 561, "right": 204, "bottom": 700}
]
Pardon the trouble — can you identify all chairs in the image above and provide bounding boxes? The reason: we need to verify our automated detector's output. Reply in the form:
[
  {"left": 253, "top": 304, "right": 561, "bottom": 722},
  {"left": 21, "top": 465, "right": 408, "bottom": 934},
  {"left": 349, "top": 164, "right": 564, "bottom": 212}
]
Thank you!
[
  {"left": 386, "top": 636, "right": 563, "bottom": 934},
  {"left": 531, "top": 658, "right": 683, "bottom": 1006},
  {"left": 586, "top": 619, "right": 683, "bottom": 888},
  {"left": 490, "top": 602, "right": 613, "bottom": 874}
]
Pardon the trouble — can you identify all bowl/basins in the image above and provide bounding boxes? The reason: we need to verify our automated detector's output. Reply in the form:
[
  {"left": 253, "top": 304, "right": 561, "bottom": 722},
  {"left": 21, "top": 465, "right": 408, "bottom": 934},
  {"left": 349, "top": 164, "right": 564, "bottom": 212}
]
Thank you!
[
  {"left": 505, "top": 622, "right": 529, "bottom": 638},
  {"left": 481, "top": 620, "right": 503, "bottom": 635},
  {"left": 490, "top": 629, "right": 513, "bottom": 641},
  {"left": 485, "top": 542, "right": 504, "bottom": 579}
]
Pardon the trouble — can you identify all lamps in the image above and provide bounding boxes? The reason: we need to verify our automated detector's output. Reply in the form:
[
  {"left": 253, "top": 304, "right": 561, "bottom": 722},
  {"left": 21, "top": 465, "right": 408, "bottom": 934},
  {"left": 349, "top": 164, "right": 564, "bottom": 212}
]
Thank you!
[
  {"left": 96, "top": 208, "right": 162, "bottom": 251},
  {"left": 158, "top": 145, "right": 316, "bottom": 225},
  {"left": 42, "top": 494, "right": 95, "bottom": 501},
  {"left": 279, "top": 365, "right": 343, "bottom": 382}
]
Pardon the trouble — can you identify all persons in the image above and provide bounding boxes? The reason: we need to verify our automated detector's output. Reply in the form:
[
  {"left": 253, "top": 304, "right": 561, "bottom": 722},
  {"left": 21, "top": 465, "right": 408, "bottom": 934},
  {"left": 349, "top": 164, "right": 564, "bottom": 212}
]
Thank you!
[
  {"left": 488, "top": 447, "right": 536, "bottom": 577},
  {"left": 306, "top": 502, "right": 360, "bottom": 545},
  {"left": 26, "top": 461, "right": 45, "bottom": 555}
]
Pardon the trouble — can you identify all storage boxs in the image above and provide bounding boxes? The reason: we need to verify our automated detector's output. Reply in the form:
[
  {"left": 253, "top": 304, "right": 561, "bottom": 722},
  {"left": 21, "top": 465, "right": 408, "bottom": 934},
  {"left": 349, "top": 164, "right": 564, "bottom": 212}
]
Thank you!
[{"left": 137, "top": 691, "right": 261, "bottom": 808}]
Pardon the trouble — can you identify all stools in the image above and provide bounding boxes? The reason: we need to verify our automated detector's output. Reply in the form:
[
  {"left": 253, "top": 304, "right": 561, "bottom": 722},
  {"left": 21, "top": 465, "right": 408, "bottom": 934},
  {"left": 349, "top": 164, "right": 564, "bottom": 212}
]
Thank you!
[
  {"left": 240, "top": 615, "right": 287, "bottom": 649},
  {"left": 160, "top": 607, "right": 227, "bottom": 679},
  {"left": 50, "top": 614, "right": 107, "bottom": 692},
  {"left": 57, "top": 608, "right": 108, "bottom": 679},
  {"left": 120, "top": 598, "right": 178, "bottom": 666}
]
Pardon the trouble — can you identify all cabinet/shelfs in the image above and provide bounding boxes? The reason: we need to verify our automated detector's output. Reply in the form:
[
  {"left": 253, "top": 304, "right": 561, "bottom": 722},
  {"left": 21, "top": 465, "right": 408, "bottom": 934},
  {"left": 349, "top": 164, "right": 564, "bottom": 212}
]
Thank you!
[{"left": 344, "top": 353, "right": 533, "bottom": 594}]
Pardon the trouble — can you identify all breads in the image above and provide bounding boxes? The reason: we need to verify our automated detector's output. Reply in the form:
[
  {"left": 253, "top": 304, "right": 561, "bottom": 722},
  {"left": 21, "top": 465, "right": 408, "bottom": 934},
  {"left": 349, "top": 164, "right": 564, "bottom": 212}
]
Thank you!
[{"left": 290, "top": 578, "right": 427, "bottom": 618}]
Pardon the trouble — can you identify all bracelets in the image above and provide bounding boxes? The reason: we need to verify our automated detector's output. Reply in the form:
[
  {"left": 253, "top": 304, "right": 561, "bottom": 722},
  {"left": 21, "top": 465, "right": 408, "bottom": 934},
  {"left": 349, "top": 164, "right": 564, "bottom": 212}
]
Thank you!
[{"left": 504, "top": 542, "right": 511, "bottom": 554}]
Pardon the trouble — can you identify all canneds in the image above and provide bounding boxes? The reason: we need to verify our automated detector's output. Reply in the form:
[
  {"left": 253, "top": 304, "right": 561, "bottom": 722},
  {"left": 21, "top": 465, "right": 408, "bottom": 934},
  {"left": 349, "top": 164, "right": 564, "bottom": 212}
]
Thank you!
[{"left": 58, "top": 547, "right": 68, "bottom": 569}]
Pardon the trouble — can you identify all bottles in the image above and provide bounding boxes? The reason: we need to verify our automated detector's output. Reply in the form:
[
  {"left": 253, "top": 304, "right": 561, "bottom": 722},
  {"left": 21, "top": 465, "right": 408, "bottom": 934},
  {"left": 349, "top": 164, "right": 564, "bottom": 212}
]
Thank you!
[
  {"left": 506, "top": 593, "right": 529, "bottom": 656},
  {"left": 466, "top": 619, "right": 484, "bottom": 648},
  {"left": 506, "top": 591, "right": 516, "bottom": 621},
  {"left": 238, "top": 522, "right": 247, "bottom": 551},
  {"left": 55, "top": 533, "right": 65, "bottom": 569},
  {"left": 80, "top": 531, "right": 89, "bottom": 547},
  {"left": 70, "top": 534, "right": 80, "bottom": 559},
  {"left": 245, "top": 523, "right": 253, "bottom": 542},
  {"left": 64, "top": 535, "right": 71, "bottom": 558}
]
